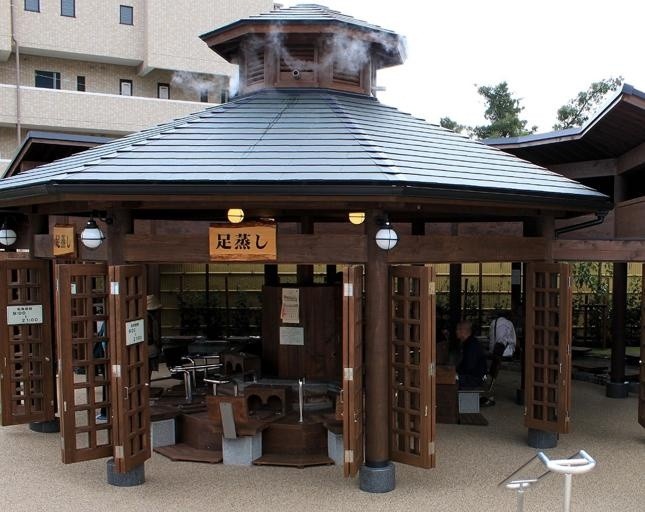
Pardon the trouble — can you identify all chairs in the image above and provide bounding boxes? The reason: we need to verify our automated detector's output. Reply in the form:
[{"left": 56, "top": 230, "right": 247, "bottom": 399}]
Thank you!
[
  {"left": 162, "top": 346, "right": 205, "bottom": 406},
  {"left": 456, "top": 343, "right": 504, "bottom": 407},
  {"left": 206, "top": 396, "right": 282, "bottom": 466}
]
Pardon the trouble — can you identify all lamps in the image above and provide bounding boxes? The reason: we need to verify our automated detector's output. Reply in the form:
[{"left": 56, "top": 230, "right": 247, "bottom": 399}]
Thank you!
[
  {"left": 346, "top": 207, "right": 366, "bottom": 224},
  {"left": 224, "top": 209, "right": 247, "bottom": 224},
  {"left": 82, "top": 213, "right": 113, "bottom": 249},
  {"left": 373, "top": 213, "right": 400, "bottom": 251},
  {"left": 0, "top": 224, "right": 18, "bottom": 246}
]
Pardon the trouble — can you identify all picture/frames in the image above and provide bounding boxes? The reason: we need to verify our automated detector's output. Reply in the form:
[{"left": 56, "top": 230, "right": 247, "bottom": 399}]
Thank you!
[
  {"left": 121, "top": 318, "right": 144, "bottom": 346},
  {"left": 5, "top": 304, "right": 44, "bottom": 326}
]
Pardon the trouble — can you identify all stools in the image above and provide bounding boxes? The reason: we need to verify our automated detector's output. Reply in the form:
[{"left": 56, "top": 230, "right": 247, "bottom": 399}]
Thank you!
[
  {"left": 138, "top": 405, "right": 178, "bottom": 448},
  {"left": 323, "top": 420, "right": 343, "bottom": 466}
]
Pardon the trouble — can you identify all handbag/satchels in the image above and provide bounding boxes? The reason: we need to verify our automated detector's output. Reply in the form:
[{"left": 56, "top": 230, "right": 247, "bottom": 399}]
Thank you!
[{"left": 493, "top": 341, "right": 506, "bottom": 358}]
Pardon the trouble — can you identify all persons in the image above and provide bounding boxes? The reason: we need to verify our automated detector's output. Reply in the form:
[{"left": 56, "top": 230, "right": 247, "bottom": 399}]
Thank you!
[
  {"left": 95, "top": 322, "right": 108, "bottom": 420},
  {"left": 487, "top": 308, "right": 516, "bottom": 358},
  {"left": 447, "top": 321, "right": 484, "bottom": 388},
  {"left": 146, "top": 295, "right": 163, "bottom": 371},
  {"left": 436, "top": 305, "right": 449, "bottom": 363}
]
